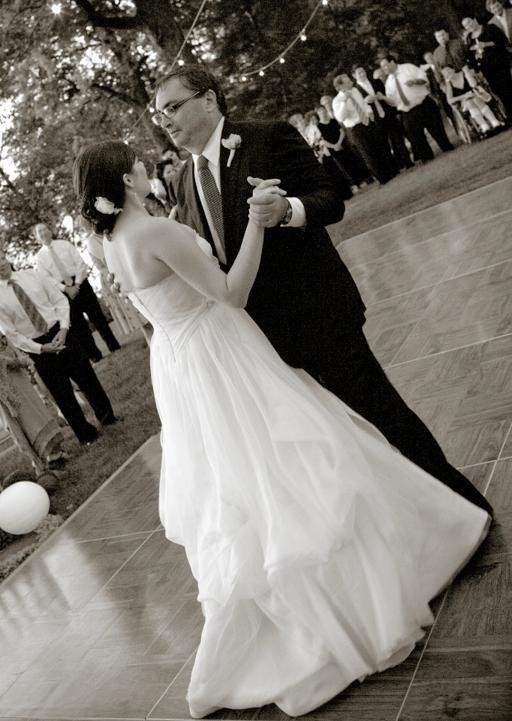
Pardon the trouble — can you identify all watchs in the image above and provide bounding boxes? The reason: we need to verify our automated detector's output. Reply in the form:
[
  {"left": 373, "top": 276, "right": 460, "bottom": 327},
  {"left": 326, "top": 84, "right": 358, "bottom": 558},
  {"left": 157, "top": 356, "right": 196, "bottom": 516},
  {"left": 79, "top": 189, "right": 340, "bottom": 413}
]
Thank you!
[{"left": 279, "top": 202, "right": 292, "bottom": 225}]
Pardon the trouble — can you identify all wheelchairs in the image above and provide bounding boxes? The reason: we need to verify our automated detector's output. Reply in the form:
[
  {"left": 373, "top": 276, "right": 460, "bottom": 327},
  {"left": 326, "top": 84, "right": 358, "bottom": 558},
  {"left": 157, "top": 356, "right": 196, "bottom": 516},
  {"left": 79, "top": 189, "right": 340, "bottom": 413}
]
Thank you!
[{"left": 438, "top": 69, "right": 509, "bottom": 145}]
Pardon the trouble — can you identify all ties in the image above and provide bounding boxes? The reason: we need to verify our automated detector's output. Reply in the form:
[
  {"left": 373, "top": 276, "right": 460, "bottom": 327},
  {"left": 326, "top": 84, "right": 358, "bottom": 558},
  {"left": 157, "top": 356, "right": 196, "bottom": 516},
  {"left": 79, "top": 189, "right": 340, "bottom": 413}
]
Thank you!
[
  {"left": 197, "top": 155, "right": 226, "bottom": 251},
  {"left": 48, "top": 246, "right": 73, "bottom": 287},
  {"left": 350, "top": 95, "right": 369, "bottom": 126},
  {"left": 394, "top": 76, "right": 409, "bottom": 106},
  {"left": 367, "top": 82, "right": 385, "bottom": 118},
  {"left": 8, "top": 279, "right": 48, "bottom": 334}
]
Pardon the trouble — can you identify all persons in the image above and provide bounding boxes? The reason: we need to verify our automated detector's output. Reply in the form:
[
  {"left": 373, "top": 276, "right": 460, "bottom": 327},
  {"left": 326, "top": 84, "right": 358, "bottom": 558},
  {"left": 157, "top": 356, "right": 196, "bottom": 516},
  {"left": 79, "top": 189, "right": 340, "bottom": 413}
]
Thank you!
[
  {"left": 151, "top": 64, "right": 495, "bottom": 517},
  {"left": 287, "top": 0, "right": 512, "bottom": 200},
  {"left": 0, "top": 147, "right": 188, "bottom": 478},
  {"left": 73, "top": 141, "right": 492, "bottom": 719}
]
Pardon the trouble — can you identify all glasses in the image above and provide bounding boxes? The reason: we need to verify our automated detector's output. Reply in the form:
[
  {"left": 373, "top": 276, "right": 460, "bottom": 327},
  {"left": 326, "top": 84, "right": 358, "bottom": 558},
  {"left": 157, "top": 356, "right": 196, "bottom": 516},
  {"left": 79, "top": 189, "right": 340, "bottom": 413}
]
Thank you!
[{"left": 151, "top": 91, "right": 201, "bottom": 125}]
